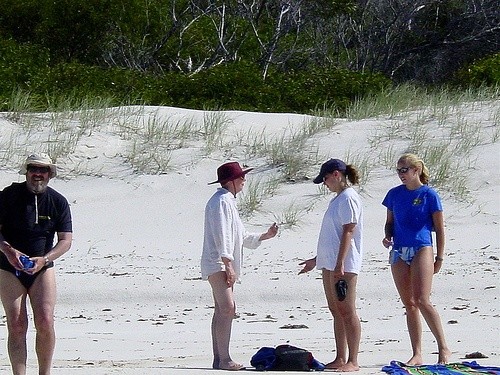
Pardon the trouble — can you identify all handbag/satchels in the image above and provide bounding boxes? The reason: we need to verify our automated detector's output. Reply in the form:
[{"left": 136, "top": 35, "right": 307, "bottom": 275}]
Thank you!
[{"left": 272, "top": 344, "right": 312, "bottom": 371}]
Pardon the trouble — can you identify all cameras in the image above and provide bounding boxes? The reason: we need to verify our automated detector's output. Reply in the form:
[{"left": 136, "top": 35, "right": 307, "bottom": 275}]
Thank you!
[{"left": 19, "top": 254, "right": 34, "bottom": 270}]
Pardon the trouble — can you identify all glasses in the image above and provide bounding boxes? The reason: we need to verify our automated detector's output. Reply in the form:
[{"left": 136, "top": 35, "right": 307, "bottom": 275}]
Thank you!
[
  {"left": 396, "top": 167, "right": 411, "bottom": 174},
  {"left": 241, "top": 176, "right": 244, "bottom": 180},
  {"left": 26, "top": 166, "right": 50, "bottom": 174},
  {"left": 323, "top": 174, "right": 332, "bottom": 181}
]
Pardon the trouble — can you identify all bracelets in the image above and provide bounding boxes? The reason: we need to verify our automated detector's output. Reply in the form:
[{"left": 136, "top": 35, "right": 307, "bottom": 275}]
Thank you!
[
  {"left": 43, "top": 256, "right": 51, "bottom": 265},
  {"left": 435, "top": 256, "right": 443, "bottom": 261}
]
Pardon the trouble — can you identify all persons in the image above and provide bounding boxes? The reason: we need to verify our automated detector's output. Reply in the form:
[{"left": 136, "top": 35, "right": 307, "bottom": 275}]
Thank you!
[
  {"left": 382, "top": 154, "right": 451, "bottom": 366},
  {"left": 297, "top": 158, "right": 363, "bottom": 372},
  {"left": 200, "top": 161, "right": 279, "bottom": 370},
  {"left": 0, "top": 152, "right": 74, "bottom": 375}
]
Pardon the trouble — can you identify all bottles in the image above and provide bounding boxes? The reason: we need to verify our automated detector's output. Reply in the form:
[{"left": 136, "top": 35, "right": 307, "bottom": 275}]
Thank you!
[{"left": 21, "top": 256, "right": 33, "bottom": 270}]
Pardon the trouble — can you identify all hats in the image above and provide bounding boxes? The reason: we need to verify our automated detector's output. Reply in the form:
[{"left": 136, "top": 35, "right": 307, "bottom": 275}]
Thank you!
[
  {"left": 313, "top": 158, "right": 347, "bottom": 184},
  {"left": 18, "top": 153, "right": 57, "bottom": 179},
  {"left": 207, "top": 162, "right": 254, "bottom": 185}
]
